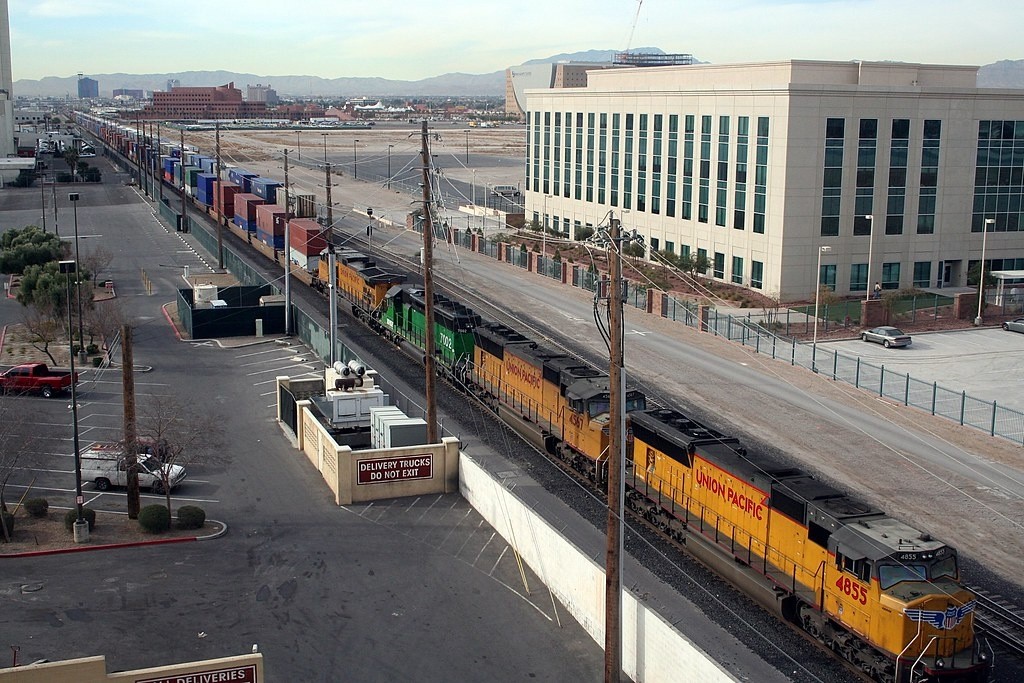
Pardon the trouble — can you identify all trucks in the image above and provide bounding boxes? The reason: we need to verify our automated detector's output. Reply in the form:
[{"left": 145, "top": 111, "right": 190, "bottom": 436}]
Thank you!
[
  {"left": 92, "top": 437, "right": 172, "bottom": 466},
  {"left": 241, "top": 107, "right": 527, "bottom": 131},
  {"left": 80, "top": 450, "right": 187, "bottom": 494}
]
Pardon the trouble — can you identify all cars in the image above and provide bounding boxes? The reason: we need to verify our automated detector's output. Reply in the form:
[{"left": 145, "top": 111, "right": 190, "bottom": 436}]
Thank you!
[
  {"left": 858, "top": 325, "right": 913, "bottom": 348},
  {"left": 34, "top": 130, "right": 99, "bottom": 161},
  {"left": 1002, "top": 317, "right": 1024, "bottom": 334}
]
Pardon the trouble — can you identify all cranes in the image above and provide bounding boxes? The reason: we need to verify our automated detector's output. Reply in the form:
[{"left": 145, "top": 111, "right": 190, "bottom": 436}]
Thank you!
[{"left": 620, "top": 1, "right": 647, "bottom": 66}]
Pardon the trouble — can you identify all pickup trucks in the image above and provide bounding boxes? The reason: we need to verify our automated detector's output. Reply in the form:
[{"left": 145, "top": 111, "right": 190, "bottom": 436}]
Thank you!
[{"left": 0, "top": 362, "right": 79, "bottom": 398}]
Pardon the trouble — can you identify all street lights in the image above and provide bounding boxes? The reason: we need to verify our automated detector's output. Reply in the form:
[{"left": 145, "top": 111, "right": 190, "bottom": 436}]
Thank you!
[
  {"left": 865, "top": 213, "right": 874, "bottom": 329},
  {"left": 975, "top": 218, "right": 996, "bottom": 327},
  {"left": 812, "top": 246, "right": 833, "bottom": 374}
]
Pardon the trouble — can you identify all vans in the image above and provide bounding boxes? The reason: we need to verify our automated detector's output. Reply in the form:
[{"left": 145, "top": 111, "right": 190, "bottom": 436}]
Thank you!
[{"left": 491, "top": 185, "right": 522, "bottom": 197}]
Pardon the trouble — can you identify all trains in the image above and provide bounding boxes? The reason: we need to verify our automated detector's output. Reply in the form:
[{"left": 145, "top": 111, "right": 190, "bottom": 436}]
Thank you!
[{"left": 317, "top": 242, "right": 999, "bottom": 683}]
